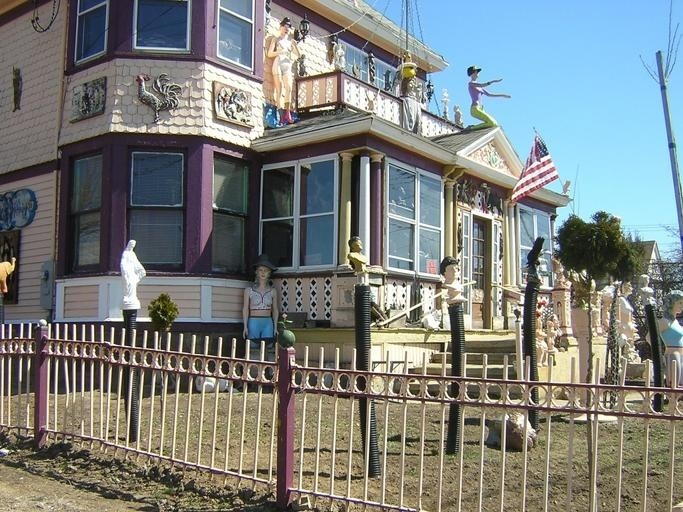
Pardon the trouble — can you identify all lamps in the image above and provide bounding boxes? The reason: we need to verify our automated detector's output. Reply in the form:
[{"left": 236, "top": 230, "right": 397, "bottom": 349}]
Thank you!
[
  {"left": 425, "top": 79, "right": 435, "bottom": 101},
  {"left": 294, "top": 12, "right": 311, "bottom": 44}
]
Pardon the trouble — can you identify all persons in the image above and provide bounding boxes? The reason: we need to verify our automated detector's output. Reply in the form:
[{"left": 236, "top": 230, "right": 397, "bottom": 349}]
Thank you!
[
  {"left": 440, "top": 255, "right": 464, "bottom": 303},
  {"left": 241, "top": 255, "right": 282, "bottom": 376},
  {"left": 329, "top": 42, "right": 347, "bottom": 76},
  {"left": 417, "top": 82, "right": 463, "bottom": 126},
  {"left": 398, "top": 76, "right": 417, "bottom": 97},
  {"left": 119, "top": 238, "right": 146, "bottom": 296},
  {"left": 348, "top": 235, "right": 368, "bottom": 270},
  {"left": 464, "top": 66, "right": 510, "bottom": 131},
  {"left": 639, "top": 273, "right": 654, "bottom": 305},
  {"left": 616, "top": 280, "right": 634, "bottom": 335},
  {"left": 535, "top": 310, "right": 562, "bottom": 366},
  {"left": 268, "top": 17, "right": 301, "bottom": 129},
  {"left": 368, "top": 53, "right": 378, "bottom": 85},
  {"left": 645, "top": 290, "right": 683, "bottom": 388}
]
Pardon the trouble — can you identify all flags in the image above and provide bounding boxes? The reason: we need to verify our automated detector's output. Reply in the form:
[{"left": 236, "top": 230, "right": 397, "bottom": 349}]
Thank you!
[{"left": 511, "top": 128, "right": 558, "bottom": 202}]
